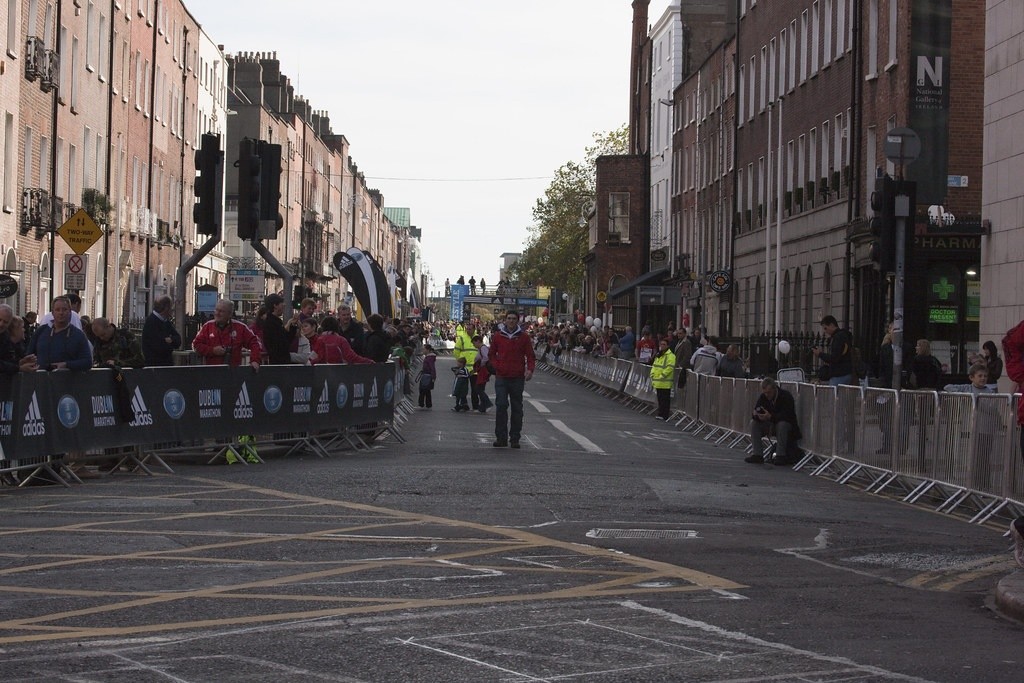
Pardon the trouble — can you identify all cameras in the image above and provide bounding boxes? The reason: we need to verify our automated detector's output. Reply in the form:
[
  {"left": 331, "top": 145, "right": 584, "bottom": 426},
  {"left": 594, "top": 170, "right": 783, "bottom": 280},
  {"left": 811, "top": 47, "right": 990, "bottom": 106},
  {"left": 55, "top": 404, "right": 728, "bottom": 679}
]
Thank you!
[
  {"left": 752, "top": 406, "right": 765, "bottom": 419},
  {"left": 222, "top": 346, "right": 232, "bottom": 365}
]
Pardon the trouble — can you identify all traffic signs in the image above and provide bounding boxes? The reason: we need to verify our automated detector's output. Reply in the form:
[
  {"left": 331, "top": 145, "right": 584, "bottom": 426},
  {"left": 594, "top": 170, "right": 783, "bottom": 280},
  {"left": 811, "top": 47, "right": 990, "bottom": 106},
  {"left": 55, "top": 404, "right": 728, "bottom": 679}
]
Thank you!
[{"left": 57, "top": 208, "right": 104, "bottom": 254}]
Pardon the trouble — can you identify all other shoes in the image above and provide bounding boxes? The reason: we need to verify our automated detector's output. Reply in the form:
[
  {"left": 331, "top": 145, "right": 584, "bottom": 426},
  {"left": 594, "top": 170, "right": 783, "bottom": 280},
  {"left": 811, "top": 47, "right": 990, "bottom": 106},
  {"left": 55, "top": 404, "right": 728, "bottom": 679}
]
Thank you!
[
  {"left": 509, "top": 438, "right": 520, "bottom": 448},
  {"left": 1010, "top": 519, "right": 1024, "bottom": 567},
  {"left": 493, "top": 439, "right": 507, "bottom": 447},
  {"left": 774, "top": 456, "right": 787, "bottom": 466},
  {"left": 99, "top": 460, "right": 139, "bottom": 472},
  {"left": 876, "top": 448, "right": 890, "bottom": 454},
  {"left": 414, "top": 406, "right": 432, "bottom": 410},
  {"left": 744, "top": 455, "right": 764, "bottom": 464}
]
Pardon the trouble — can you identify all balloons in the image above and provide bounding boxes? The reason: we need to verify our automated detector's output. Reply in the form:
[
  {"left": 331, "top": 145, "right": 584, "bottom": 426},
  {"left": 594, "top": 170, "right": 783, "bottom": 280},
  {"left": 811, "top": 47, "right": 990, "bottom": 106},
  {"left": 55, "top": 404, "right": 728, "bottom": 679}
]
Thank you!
[
  {"left": 593, "top": 318, "right": 602, "bottom": 328},
  {"left": 541, "top": 309, "right": 549, "bottom": 317},
  {"left": 414, "top": 308, "right": 419, "bottom": 314},
  {"left": 590, "top": 326, "right": 597, "bottom": 333},
  {"left": 585, "top": 316, "right": 594, "bottom": 326}
]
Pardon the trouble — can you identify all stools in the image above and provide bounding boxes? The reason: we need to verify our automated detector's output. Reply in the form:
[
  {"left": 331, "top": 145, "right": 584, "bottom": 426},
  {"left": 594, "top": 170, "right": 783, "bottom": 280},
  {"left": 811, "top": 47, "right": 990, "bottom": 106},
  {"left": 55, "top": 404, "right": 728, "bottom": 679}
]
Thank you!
[{"left": 765, "top": 433, "right": 802, "bottom": 460}]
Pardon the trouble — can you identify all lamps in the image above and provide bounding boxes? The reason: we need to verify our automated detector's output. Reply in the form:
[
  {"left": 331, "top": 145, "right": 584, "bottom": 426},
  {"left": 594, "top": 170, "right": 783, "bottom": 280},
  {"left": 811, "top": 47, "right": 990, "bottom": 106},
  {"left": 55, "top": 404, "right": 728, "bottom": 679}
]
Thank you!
[
  {"left": 965, "top": 263, "right": 978, "bottom": 276},
  {"left": 577, "top": 201, "right": 594, "bottom": 227},
  {"left": 388, "top": 222, "right": 405, "bottom": 244},
  {"left": 348, "top": 194, "right": 370, "bottom": 224}
]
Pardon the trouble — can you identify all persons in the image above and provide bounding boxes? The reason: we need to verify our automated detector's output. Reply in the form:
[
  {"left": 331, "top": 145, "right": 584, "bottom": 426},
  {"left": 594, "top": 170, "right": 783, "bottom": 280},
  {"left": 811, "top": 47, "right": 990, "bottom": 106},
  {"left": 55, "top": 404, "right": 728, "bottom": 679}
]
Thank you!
[
  {"left": 190, "top": 275, "right": 748, "bottom": 489},
  {"left": 876, "top": 319, "right": 1003, "bottom": 498},
  {"left": 488, "top": 310, "right": 536, "bottom": 449},
  {"left": 415, "top": 344, "right": 437, "bottom": 411},
  {"left": 452, "top": 323, "right": 485, "bottom": 413},
  {"left": 470, "top": 335, "right": 493, "bottom": 413},
  {"left": 810, "top": 315, "right": 867, "bottom": 455},
  {"left": 1, "top": 293, "right": 183, "bottom": 488},
  {"left": 448, "top": 356, "right": 471, "bottom": 412},
  {"left": 649, "top": 339, "right": 677, "bottom": 420},
  {"left": 1002, "top": 318, "right": 1023, "bottom": 569},
  {"left": 744, "top": 377, "right": 803, "bottom": 466}
]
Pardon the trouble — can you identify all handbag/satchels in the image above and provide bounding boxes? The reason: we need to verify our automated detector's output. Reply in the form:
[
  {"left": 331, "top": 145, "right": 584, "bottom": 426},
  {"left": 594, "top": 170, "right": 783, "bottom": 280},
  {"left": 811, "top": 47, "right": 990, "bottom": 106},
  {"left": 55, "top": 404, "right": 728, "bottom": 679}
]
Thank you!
[{"left": 486, "top": 361, "right": 495, "bottom": 374}]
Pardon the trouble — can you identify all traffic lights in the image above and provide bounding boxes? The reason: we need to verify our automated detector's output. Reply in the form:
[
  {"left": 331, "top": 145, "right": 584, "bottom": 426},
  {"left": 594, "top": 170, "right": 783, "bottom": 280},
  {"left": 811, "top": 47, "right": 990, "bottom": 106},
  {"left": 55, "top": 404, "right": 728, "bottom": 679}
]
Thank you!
[
  {"left": 193, "top": 132, "right": 220, "bottom": 235},
  {"left": 234, "top": 137, "right": 283, "bottom": 241},
  {"left": 292, "top": 285, "right": 302, "bottom": 309},
  {"left": 868, "top": 174, "right": 896, "bottom": 280}
]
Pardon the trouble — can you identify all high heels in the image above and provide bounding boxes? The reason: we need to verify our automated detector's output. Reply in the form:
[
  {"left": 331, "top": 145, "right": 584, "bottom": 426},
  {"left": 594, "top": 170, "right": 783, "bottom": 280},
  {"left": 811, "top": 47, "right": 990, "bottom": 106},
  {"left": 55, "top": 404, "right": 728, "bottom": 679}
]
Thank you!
[{"left": 1, "top": 460, "right": 17, "bottom": 486}]
